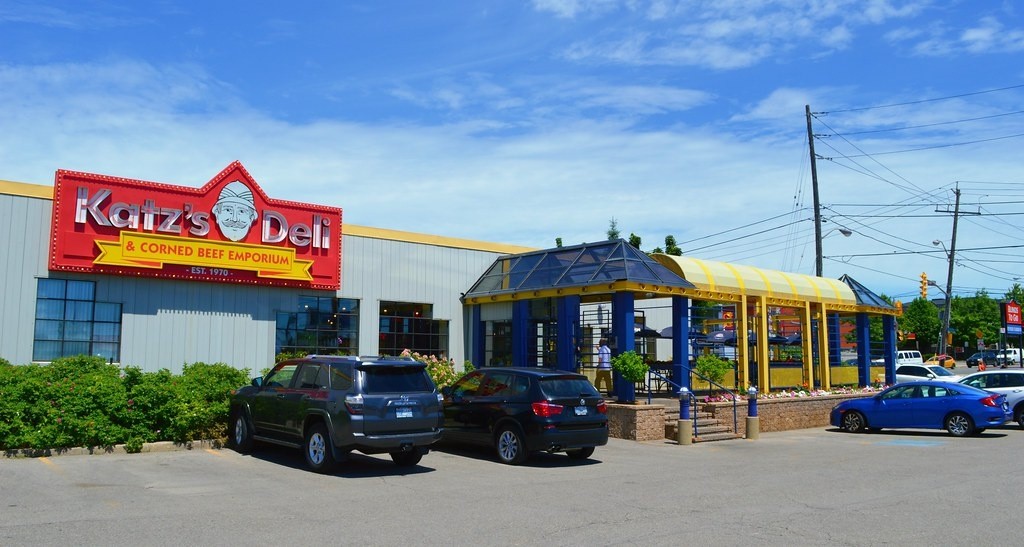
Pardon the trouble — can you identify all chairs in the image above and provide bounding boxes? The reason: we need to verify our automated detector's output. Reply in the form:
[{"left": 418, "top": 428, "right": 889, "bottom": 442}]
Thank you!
[
  {"left": 301, "top": 367, "right": 318, "bottom": 389},
  {"left": 912, "top": 388, "right": 923, "bottom": 398}
]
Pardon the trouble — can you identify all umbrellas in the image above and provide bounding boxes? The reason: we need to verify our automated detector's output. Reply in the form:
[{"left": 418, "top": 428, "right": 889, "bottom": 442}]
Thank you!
[{"left": 603, "top": 323, "right": 802, "bottom": 346}]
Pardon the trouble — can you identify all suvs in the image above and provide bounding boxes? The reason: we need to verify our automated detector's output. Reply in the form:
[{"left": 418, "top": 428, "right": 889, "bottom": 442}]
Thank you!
[{"left": 227, "top": 354, "right": 445, "bottom": 473}]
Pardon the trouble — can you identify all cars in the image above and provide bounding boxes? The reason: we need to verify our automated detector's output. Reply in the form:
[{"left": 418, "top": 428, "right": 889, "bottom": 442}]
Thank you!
[
  {"left": 924, "top": 355, "right": 956, "bottom": 370},
  {"left": 879, "top": 364, "right": 960, "bottom": 384},
  {"left": 438, "top": 366, "right": 609, "bottom": 466},
  {"left": 922, "top": 370, "right": 1024, "bottom": 430},
  {"left": 829, "top": 379, "right": 1015, "bottom": 437},
  {"left": 966, "top": 351, "right": 1000, "bottom": 368}
]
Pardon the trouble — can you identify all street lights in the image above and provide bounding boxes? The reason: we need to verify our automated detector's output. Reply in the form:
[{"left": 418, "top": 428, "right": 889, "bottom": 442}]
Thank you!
[
  {"left": 815, "top": 227, "right": 852, "bottom": 278},
  {"left": 932, "top": 239, "right": 955, "bottom": 356}
]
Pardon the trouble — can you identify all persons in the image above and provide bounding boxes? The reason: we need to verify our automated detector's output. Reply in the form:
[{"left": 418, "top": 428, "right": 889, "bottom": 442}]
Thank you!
[
  {"left": 594, "top": 338, "right": 613, "bottom": 398},
  {"left": 976, "top": 358, "right": 986, "bottom": 372}
]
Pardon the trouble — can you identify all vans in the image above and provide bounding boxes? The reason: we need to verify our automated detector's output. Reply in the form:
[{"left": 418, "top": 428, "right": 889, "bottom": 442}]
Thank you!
[
  {"left": 895, "top": 350, "right": 923, "bottom": 371},
  {"left": 996, "top": 348, "right": 1024, "bottom": 365}
]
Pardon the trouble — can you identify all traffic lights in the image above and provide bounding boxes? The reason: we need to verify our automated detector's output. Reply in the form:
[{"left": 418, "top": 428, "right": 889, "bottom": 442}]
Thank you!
[
  {"left": 896, "top": 330, "right": 905, "bottom": 341},
  {"left": 919, "top": 272, "right": 928, "bottom": 298}
]
